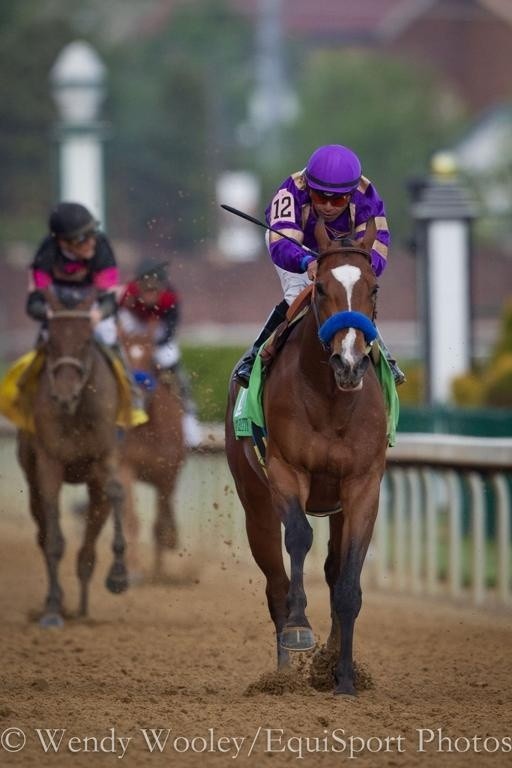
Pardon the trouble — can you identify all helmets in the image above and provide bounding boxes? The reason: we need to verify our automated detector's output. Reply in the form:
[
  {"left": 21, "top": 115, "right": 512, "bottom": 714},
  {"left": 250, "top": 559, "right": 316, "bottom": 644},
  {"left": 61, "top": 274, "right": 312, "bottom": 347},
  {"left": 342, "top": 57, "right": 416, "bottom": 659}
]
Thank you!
[
  {"left": 49, "top": 203, "right": 98, "bottom": 240},
  {"left": 303, "top": 144, "right": 362, "bottom": 194}
]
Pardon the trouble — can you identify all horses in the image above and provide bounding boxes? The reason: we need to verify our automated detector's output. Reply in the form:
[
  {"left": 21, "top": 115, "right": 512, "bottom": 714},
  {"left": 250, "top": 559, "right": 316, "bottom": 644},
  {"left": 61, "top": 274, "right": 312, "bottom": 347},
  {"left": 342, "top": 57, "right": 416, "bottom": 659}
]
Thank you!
[
  {"left": 17, "top": 284, "right": 128, "bottom": 627},
  {"left": 224, "top": 215, "right": 388, "bottom": 701},
  {"left": 115, "top": 312, "right": 187, "bottom": 584}
]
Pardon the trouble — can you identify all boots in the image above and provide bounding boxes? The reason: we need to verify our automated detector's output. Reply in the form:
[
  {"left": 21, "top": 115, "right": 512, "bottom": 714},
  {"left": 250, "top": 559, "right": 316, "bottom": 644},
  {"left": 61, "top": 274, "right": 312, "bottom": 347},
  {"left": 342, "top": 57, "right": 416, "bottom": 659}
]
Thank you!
[
  {"left": 232, "top": 304, "right": 286, "bottom": 389},
  {"left": 373, "top": 320, "right": 405, "bottom": 386}
]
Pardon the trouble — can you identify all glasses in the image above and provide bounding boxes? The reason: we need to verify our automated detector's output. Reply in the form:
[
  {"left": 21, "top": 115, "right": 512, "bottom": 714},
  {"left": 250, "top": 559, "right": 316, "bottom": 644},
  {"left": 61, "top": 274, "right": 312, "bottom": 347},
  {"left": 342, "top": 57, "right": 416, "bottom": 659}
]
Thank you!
[
  {"left": 308, "top": 186, "right": 355, "bottom": 207},
  {"left": 64, "top": 227, "right": 99, "bottom": 248}
]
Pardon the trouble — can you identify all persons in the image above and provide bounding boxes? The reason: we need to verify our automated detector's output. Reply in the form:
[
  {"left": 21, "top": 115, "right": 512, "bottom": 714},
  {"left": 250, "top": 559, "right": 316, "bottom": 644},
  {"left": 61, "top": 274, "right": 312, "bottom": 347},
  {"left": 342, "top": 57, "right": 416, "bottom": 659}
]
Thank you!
[
  {"left": 94, "top": 260, "right": 207, "bottom": 452},
  {"left": 13, "top": 201, "right": 151, "bottom": 419},
  {"left": 231, "top": 142, "right": 407, "bottom": 390}
]
